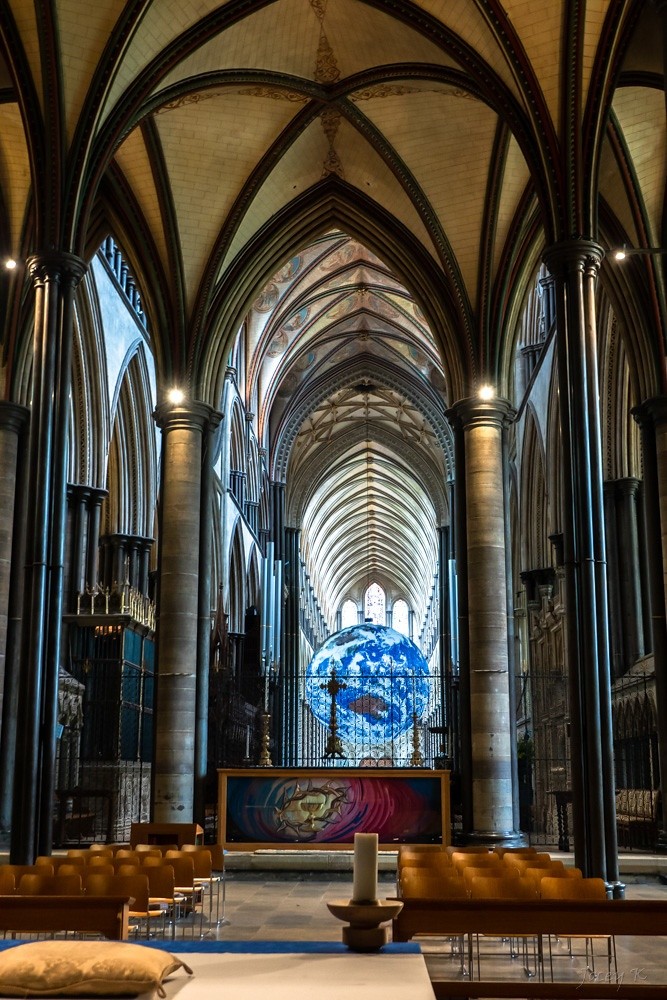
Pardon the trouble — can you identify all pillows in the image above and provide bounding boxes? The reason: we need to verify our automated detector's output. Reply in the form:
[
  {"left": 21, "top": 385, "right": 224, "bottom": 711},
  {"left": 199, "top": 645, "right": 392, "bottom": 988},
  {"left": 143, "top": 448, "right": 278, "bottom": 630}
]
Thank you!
[{"left": 0, "top": 940, "right": 193, "bottom": 998}]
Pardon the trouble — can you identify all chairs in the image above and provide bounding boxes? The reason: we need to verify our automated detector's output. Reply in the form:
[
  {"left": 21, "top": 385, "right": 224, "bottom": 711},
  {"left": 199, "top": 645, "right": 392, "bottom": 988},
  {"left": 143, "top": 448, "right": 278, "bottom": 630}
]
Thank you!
[
  {"left": 396, "top": 845, "right": 619, "bottom": 984},
  {"left": 0, "top": 844, "right": 226, "bottom": 940}
]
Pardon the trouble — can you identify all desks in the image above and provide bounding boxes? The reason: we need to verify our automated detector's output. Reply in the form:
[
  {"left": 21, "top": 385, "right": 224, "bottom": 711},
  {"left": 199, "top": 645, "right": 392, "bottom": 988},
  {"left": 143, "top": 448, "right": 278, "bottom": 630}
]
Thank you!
[{"left": 0, "top": 940, "right": 436, "bottom": 1000}]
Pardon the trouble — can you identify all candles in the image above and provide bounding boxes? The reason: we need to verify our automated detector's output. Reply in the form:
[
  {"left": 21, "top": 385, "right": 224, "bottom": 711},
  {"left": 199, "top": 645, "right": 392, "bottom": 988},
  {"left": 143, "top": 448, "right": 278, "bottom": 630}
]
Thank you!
[{"left": 352, "top": 831, "right": 379, "bottom": 901}]
